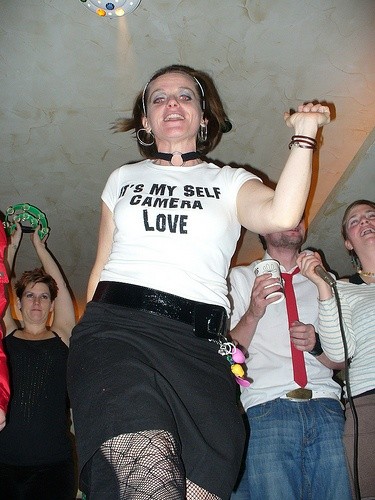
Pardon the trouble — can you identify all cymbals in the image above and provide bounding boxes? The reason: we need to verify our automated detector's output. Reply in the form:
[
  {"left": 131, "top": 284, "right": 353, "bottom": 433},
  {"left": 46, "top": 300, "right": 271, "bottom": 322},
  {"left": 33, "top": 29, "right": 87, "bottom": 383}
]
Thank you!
[{"left": 2, "top": 203, "right": 49, "bottom": 239}]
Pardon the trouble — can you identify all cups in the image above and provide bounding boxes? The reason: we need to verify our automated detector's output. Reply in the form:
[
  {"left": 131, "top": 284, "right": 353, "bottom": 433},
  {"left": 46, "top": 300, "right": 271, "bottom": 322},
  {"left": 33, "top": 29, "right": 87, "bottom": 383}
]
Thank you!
[{"left": 253, "top": 259, "right": 285, "bottom": 305}]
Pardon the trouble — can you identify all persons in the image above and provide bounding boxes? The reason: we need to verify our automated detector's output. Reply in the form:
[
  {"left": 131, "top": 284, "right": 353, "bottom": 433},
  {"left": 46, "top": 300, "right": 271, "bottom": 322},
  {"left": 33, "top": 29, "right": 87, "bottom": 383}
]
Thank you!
[
  {"left": 296, "top": 199, "right": 375, "bottom": 500},
  {"left": 226, "top": 213, "right": 352, "bottom": 500},
  {"left": 0, "top": 218, "right": 78, "bottom": 500},
  {"left": 66, "top": 65, "right": 331, "bottom": 500}
]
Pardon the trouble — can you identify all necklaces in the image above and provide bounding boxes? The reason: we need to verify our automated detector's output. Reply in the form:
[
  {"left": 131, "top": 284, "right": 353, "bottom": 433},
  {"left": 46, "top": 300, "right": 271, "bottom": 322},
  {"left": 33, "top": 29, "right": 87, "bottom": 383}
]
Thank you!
[
  {"left": 154, "top": 151, "right": 202, "bottom": 167},
  {"left": 358, "top": 269, "right": 375, "bottom": 278}
]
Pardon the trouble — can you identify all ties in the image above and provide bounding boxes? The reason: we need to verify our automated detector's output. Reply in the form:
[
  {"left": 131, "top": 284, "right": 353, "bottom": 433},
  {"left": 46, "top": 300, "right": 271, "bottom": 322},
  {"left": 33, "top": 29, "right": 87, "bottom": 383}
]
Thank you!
[{"left": 280, "top": 269, "right": 309, "bottom": 386}]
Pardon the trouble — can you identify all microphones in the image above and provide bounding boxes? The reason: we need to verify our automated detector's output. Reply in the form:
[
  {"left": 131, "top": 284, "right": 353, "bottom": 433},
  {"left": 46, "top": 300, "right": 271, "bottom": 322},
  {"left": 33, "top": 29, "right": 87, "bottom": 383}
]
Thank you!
[{"left": 301, "top": 249, "right": 336, "bottom": 286}]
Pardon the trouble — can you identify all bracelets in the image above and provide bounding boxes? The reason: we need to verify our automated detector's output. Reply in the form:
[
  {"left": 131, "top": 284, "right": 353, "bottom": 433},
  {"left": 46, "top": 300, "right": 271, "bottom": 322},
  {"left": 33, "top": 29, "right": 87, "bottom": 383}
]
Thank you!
[
  {"left": 288, "top": 136, "right": 318, "bottom": 152},
  {"left": 309, "top": 332, "right": 323, "bottom": 357},
  {"left": 317, "top": 294, "right": 334, "bottom": 302}
]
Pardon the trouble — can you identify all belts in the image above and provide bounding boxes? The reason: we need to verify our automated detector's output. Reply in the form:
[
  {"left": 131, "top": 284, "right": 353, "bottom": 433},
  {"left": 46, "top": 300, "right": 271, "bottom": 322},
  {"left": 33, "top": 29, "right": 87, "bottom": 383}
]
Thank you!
[
  {"left": 285, "top": 388, "right": 312, "bottom": 400},
  {"left": 93, "top": 281, "right": 228, "bottom": 339}
]
